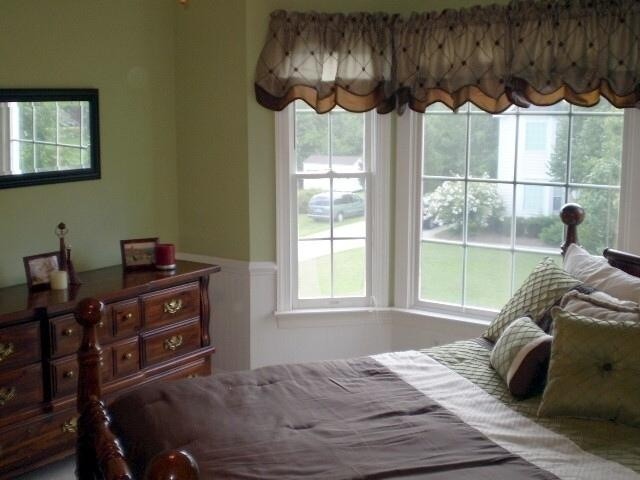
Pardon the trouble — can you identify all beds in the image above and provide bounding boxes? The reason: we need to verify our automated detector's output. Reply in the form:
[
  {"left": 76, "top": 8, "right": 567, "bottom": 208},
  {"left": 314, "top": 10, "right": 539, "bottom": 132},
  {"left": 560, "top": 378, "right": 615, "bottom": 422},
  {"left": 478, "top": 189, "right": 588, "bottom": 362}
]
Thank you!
[{"left": 76, "top": 202, "right": 640, "bottom": 478}]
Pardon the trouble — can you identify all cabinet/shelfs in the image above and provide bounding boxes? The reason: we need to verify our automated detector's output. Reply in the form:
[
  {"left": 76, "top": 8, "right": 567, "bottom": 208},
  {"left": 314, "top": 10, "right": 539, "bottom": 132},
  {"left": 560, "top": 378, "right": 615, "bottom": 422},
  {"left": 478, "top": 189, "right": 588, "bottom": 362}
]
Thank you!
[{"left": 1, "top": 259, "right": 219, "bottom": 479}]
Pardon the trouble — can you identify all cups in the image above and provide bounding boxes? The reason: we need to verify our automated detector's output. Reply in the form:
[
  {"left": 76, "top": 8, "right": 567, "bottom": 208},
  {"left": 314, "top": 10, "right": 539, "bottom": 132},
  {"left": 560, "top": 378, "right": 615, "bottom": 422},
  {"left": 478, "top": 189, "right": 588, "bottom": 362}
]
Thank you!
[{"left": 153, "top": 243, "right": 176, "bottom": 271}]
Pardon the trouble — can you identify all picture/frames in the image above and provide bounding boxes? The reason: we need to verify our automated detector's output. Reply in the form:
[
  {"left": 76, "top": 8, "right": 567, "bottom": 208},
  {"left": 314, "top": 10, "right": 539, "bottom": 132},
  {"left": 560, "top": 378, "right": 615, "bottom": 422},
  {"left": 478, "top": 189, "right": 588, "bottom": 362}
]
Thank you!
[
  {"left": 22, "top": 252, "right": 63, "bottom": 295},
  {"left": 120, "top": 237, "right": 160, "bottom": 275}
]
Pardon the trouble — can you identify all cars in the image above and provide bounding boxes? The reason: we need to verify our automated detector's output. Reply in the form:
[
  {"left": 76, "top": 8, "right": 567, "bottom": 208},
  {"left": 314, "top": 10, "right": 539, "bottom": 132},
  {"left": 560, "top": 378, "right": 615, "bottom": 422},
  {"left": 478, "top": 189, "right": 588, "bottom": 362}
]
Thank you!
[{"left": 307, "top": 191, "right": 364, "bottom": 223}]
